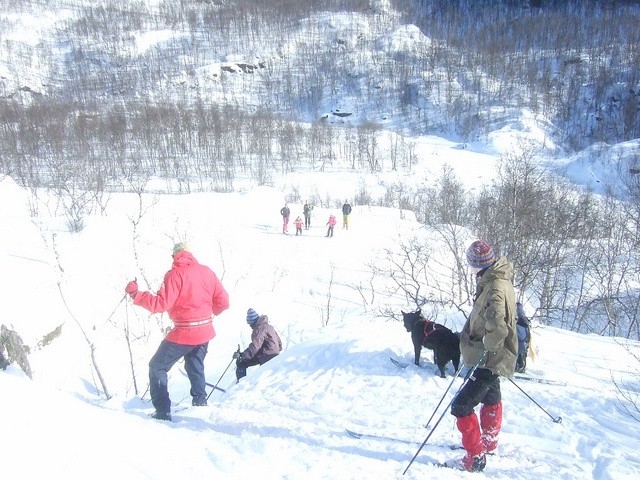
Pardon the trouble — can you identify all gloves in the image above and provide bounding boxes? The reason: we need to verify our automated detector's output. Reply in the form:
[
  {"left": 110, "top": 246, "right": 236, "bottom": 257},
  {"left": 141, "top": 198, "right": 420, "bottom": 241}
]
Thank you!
[
  {"left": 125, "top": 280, "right": 138, "bottom": 298},
  {"left": 232, "top": 350, "right": 241, "bottom": 360}
]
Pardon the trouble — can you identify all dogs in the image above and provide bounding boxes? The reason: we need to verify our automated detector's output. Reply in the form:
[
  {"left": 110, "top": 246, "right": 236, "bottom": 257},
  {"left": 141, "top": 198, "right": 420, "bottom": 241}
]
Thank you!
[{"left": 403, "top": 310, "right": 461, "bottom": 378}]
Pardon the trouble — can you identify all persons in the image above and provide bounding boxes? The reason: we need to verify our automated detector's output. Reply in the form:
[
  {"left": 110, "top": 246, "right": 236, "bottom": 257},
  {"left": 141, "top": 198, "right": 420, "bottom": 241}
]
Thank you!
[
  {"left": 303, "top": 200, "right": 312, "bottom": 230},
  {"left": 515, "top": 300, "right": 531, "bottom": 373},
  {"left": 342, "top": 199, "right": 351, "bottom": 229},
  {"left": 125, "top": 241, "right": 229, "bottom": 421},
  {"left": 293, "top": 216, "right": 304, "bottom": 236},
  {"left": 233, "top": 308, "right": 282, "bottom": 383},
  {"left": 281, "top": 203, "right": 290, "bottom": 235},
  {"left": 325, "top": 214, "right": 336, "bottom": 237},
  {"left": 450, "top": 240, "right": 519, "bottom": 473}
]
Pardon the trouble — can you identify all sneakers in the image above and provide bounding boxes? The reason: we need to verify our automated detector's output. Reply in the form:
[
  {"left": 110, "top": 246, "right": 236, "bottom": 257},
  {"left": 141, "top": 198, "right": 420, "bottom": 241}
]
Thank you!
[
  {"left": 191, "top": 399, "right": 208, "bottom": 407},
  {"left": 444, "top": 452, "right": 486, "bottom": 472},
  {"left": 150, "top": 410, "right": 171, "bottom": 421}
]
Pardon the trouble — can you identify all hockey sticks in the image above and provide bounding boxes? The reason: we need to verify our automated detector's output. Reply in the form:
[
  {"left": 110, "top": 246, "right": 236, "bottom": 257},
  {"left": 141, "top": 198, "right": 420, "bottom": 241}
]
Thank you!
[
  {"left": 391, "top": 355, "right": 568, "bottom": 386},
  {"left": 179, "top": 365, "right": 227, "bottom": 392},
  {"left": 346, "top": 429, "right": 498, "bottom": 475}
]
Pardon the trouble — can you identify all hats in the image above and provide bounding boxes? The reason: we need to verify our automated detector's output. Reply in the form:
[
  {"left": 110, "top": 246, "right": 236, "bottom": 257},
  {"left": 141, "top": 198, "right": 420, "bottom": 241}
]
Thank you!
[
  {"left": 246, "top": 308, "right": 259, "bottom": 324},
  {"left": 466, "top": 240, "right": 496, "bottom": 269},
  {"left": 172, "top": 242, "right": 188, "bottom": 257}
]
